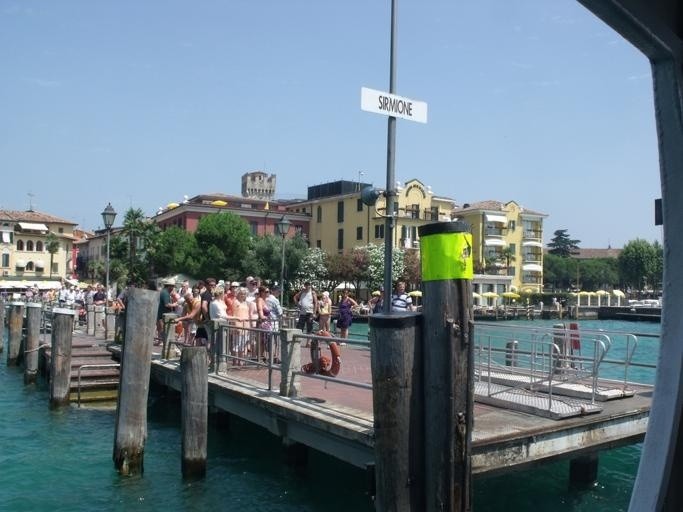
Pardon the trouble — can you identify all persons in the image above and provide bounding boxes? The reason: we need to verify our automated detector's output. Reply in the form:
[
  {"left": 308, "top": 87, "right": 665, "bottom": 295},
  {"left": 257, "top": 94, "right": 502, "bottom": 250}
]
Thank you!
[
  {"left": 10, "top": 275, "right": 136, "bottom": 345},
  {"left": 338, "top": 290, "right": 358, "bottom": 348},
  {"left": 145, "top": 276, "right": 341, "bottom": 377},
  {"left": 390, "top": 282, "right": 414, "bottom": 315},
  {"left": 366, "top": 291, "right": 385, "bottom": 319}
]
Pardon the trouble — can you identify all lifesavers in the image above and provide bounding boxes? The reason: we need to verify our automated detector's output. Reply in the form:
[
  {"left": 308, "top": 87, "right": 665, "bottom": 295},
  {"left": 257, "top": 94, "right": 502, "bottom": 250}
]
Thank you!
[{"left": 310, "top": 331, "right": 341, "bottom": 377}]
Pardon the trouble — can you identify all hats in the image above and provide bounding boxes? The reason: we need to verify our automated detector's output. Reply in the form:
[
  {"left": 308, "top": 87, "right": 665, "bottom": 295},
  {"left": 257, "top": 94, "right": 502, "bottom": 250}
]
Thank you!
[
  {"left": 230, "top": 281, "right": 240, "bottom": 287},
  {"left": 162, "top": 278, "right": 176, "bottom": 286},
  {"left": 303, "top": 281, "right": 311, "bottom": 285},
  {"left": 321, "top": 291, "right": 330, "bottom": 296},
  {"left": 245, "top": 275, "right": 254, "bottom": 286}
]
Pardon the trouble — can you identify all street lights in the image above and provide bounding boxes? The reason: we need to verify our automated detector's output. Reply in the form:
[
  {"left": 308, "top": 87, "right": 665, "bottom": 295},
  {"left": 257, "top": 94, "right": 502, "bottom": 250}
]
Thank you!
[
  {"left": 100, "top": 202, "right": 116, "bottom": 306},
  {"left": 275, "top": 212, "right": 292, "bottom": 317}
]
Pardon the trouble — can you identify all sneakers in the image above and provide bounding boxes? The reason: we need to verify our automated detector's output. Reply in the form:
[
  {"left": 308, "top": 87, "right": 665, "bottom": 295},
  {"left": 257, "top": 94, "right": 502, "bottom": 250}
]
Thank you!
[{"left": 338, "top": 342, "right": 348, "bottom": 346}]
